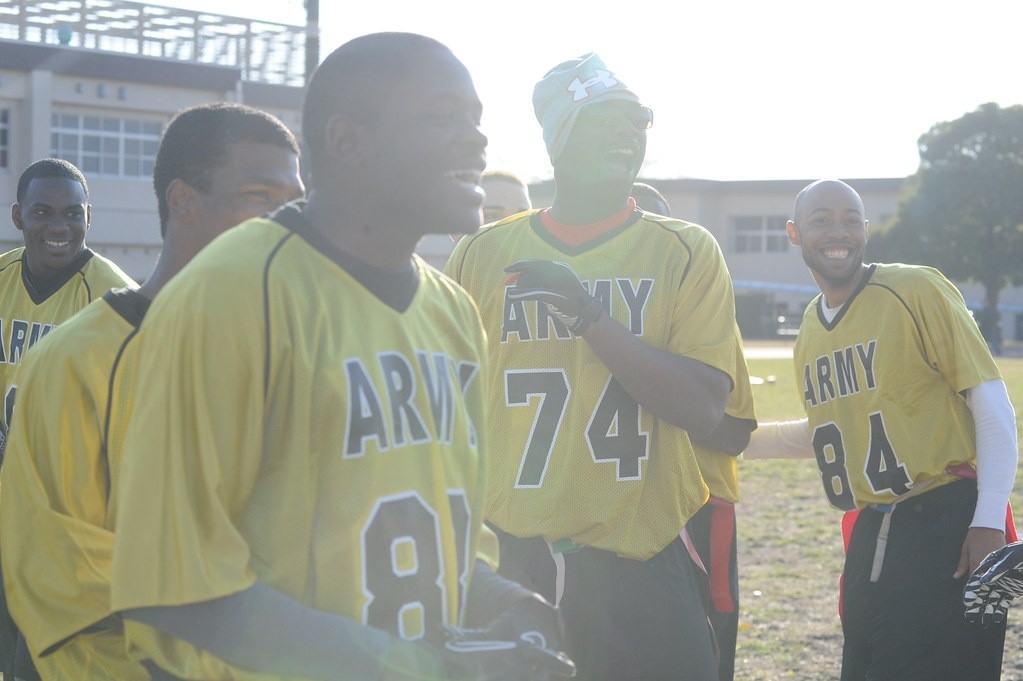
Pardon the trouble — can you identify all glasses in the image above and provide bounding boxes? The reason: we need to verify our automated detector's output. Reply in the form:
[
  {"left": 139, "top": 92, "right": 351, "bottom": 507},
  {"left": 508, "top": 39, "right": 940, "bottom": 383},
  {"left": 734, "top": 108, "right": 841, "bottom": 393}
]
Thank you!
[
  {"left": 172, "top": 169, "right": 306, "bottom": 214},
  {"left": 585, "top": 107, "right": 654, "bottom": 129}
]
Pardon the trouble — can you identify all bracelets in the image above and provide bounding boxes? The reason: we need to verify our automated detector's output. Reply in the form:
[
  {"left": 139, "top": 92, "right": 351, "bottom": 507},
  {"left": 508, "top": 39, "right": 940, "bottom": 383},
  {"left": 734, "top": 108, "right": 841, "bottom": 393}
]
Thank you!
[{"left": 569, "top": 298, "right": 604, "bottom": 337}]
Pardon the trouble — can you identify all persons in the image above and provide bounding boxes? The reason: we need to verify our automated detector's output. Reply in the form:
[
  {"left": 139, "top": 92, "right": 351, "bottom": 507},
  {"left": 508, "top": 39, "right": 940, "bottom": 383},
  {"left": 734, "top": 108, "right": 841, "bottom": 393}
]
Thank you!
[
  {"left": 0, "top": 34, "right": 758, "bottom": 680},
  {"left": 746, "top": 180, "right": 1017, "bottom": 681}
]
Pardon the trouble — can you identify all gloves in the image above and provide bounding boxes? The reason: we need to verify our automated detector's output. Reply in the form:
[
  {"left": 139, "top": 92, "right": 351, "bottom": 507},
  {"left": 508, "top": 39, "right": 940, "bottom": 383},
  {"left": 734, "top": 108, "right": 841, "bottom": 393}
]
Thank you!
[
  {"left": 502, "top": 258, "right": 606, "bottom": 336},
  {"left": 443, "top": 588, "right": 577, "bottom": 681},
  {"left": 962, "top": 541, "right": 1023, "bottom": 632}
]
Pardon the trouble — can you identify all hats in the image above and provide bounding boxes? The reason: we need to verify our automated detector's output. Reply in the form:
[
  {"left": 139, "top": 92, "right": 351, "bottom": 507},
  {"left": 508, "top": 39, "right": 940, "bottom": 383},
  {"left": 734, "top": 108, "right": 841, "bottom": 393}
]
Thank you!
[
  {"left": 532, "top": 51, "right": 639, "bottom": 161},
  {"left": 631, "top": 182, "right": 671, "bottom": 217}
]
